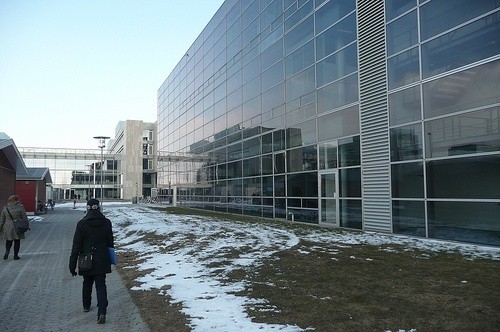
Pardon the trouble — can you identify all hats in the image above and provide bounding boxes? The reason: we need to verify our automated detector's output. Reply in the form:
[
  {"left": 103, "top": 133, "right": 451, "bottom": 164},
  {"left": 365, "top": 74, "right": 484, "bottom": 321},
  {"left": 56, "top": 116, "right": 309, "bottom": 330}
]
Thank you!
[{"left": 87, "top": 199, "right": 99, "bottom": 209}]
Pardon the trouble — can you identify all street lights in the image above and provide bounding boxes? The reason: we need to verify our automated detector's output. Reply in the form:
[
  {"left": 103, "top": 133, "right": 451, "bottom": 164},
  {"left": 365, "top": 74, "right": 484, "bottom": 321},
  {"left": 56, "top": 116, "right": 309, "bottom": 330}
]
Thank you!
[{"left": 93, "top": 135, "right": 110, "bottom": 206}]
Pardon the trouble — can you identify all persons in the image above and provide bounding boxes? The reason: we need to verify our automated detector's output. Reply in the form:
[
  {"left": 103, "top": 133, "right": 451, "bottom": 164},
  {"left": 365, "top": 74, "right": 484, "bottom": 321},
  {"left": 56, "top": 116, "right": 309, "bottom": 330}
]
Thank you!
[
  {"left": 0, "top": 195, "right": 29, "bottom": 260},
  {"left": 78, "top": 194, "right": 80, "bottom": 200},
  {"left": 69, "top": 199, "right": 114, "bottom": 325},
  {"left": 74, "top": 194, "right": 77, "bottom": 200}
]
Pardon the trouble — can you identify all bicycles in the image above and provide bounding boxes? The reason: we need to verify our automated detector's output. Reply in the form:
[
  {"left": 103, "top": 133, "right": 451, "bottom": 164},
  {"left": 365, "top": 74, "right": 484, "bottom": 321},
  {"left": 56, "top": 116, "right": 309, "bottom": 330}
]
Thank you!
[{"left": 41, "top": 199, "right": 56, "bottom": 214}]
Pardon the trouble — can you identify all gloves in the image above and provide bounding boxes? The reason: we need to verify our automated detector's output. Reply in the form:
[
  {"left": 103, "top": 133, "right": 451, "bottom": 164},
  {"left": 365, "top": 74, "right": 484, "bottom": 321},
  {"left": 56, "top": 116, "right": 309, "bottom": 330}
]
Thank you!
[{"left": 70, "top": 269, "right": 77, "bottom": 276}]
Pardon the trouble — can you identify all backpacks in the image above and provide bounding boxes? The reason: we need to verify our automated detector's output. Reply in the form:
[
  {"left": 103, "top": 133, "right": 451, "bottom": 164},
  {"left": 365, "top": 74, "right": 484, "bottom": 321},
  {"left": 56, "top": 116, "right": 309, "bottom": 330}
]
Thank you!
[{"left": 78, "top": 245, "right": 93, "bottom": 272}]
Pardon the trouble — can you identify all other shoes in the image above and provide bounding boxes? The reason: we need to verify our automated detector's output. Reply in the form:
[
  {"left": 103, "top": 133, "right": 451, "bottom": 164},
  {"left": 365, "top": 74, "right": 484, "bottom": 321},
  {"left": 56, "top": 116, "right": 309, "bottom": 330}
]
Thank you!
[
  {"left": 14, "top": 255, "right": 20, "bottom": 260},
  {"left": 84, "top": 307, "right": 88, "bottom": 311},
  {"left": 97, "top": 314, "right": 105, "bottom": 323},
  {"left": 4, "top": 255, "right": 8, "bottom": 259}
]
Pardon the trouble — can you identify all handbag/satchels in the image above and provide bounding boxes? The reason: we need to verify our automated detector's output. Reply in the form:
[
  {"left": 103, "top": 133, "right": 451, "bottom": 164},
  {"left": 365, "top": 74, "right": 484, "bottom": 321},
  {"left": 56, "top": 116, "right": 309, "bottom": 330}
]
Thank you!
[{"left": 13, "top": 219, "right": 31, "bottom": 234}]
